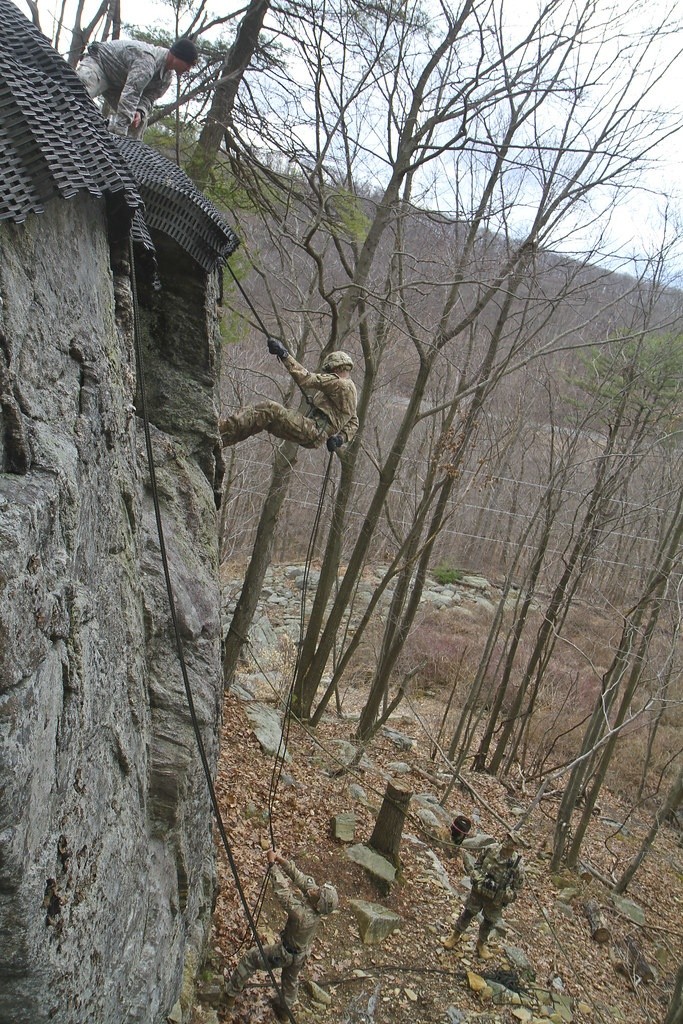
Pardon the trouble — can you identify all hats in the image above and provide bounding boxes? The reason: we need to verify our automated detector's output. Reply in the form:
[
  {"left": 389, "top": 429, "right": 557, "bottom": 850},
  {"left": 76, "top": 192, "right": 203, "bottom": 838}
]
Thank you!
[
  {"left": 170, "top": 39, "right": 198, "bottom": 66},
  {"left": 507, "top": 830, "right": 520, "bottom": 845}
]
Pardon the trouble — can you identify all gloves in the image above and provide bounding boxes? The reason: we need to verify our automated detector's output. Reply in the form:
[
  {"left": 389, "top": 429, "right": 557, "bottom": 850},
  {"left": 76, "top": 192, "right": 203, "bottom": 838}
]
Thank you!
[
  {"left": 327, "top": 435, "right": 343, "bottom": 452},
  {"left": 268, "top": 337, "right": 288, "bottom": 363}
]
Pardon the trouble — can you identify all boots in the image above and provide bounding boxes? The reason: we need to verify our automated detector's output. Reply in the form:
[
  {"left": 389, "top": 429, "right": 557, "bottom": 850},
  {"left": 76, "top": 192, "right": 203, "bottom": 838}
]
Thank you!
[
  {"left": 213, "top": 990, "right": 235, "bottom": 1024},
  {"left": 444, "top": 930, "right": 460, "bottom": 949},
  {"left": 476, "top": 938, "right": 492, "bottom": 960},
  {"left": 272, "top": 1000, "right": 290, "bottom": 1023}
]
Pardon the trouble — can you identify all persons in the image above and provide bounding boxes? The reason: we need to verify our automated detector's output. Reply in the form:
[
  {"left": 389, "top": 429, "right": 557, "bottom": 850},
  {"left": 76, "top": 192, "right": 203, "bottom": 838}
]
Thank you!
[
  {"left": 76, "top": 38, "right": 198, "bottom": 139},
  {"left": 442, "top": 830, "right": 523, "bottom": 959},
  {"left": 218, "top": 849, "right": 338, "bottom": 1024},
  {"left": 220, "top": 338, "right": 358, "bottom": 448}
]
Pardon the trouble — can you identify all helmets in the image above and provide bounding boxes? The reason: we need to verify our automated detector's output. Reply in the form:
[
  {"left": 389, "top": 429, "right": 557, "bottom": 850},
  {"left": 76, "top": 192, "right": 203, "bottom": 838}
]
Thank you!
[
  {"left": 316, "top": 884, "right": 338, "bottom": 914},
  {"left": 321, "top": 351, "right": 353, "bottom": 373}
]
